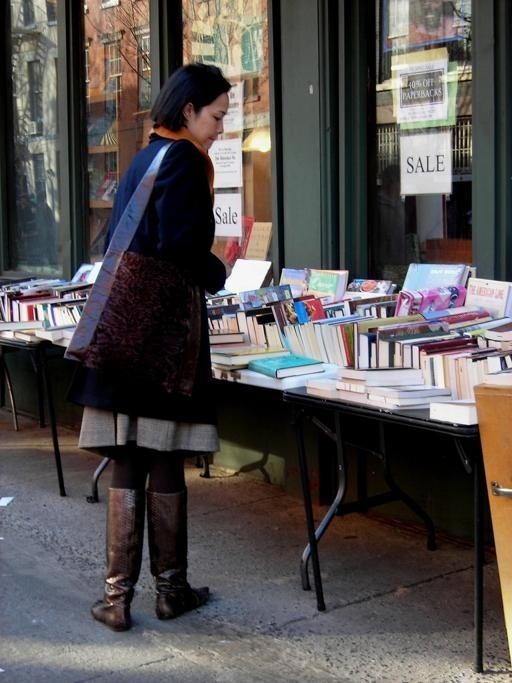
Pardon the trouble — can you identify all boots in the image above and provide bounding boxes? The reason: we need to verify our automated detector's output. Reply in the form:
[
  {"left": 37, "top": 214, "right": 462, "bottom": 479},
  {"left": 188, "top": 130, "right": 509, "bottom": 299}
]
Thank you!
[
  {"left": 91, "top": 486, "right": 146, "bottom": 632},
  {"left": 146, "top": 484, "right": 210, "bottom": 620}
]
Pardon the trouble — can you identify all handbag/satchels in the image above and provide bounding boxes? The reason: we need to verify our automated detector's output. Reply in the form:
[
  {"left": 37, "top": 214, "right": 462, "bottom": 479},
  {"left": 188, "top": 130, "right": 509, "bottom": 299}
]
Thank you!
[{"left": 62, "top": 248, "right": 202, "bottom": 397}]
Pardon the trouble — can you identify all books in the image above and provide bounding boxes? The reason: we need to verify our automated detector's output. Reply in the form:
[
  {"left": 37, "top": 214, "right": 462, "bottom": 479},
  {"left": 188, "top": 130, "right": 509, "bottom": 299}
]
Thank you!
[
  {"left": 1, "top": 261, "right": 104, "bottom": 347},
  {"left": 204, "top": 260, "right": 512, "bottom": 425}
]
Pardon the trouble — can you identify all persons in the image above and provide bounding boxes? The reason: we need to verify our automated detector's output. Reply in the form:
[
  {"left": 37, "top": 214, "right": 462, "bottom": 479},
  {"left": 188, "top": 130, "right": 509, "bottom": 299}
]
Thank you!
[
  {"left": 411, "top": 0, "right": 460, "bottom": 45},
  {"left": 61, "top": 59, "right": 236, "bottom": 632}
]
